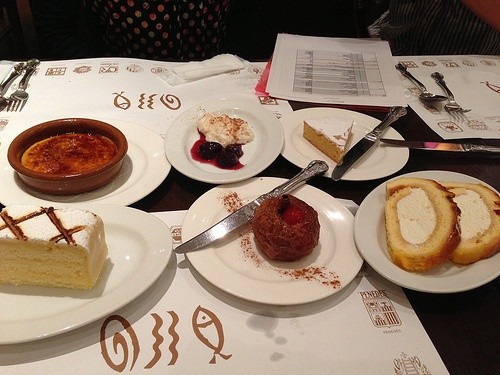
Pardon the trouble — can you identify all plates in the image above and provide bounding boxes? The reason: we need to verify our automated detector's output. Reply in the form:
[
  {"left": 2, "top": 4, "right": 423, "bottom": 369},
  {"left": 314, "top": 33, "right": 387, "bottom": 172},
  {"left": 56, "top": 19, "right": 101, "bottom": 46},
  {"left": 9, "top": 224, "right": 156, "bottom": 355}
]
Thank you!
[
  {"left": 181, "top": 177, "right": 364, "bottom": 306},
  {"left": 352, "top": 169, "right": 500, "bottom": 293},
  {"left": 278, "top": 107, "right": 410, "bottom": 181},
  {"left": 1, "top": 203, "right": 174, "bottom": 344},
  {"left": 163, "top": 97, "right": 285, "bottom": 185},
  {"left": 1, "top": 115, "right": 171, "bottom": 205}
]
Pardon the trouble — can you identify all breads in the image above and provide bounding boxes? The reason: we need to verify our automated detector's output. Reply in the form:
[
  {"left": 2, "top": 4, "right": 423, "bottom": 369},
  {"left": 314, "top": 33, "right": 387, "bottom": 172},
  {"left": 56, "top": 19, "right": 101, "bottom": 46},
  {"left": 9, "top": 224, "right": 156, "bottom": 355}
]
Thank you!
[{"left": 0, "top": 205, "right": 108, "bottom": 290}]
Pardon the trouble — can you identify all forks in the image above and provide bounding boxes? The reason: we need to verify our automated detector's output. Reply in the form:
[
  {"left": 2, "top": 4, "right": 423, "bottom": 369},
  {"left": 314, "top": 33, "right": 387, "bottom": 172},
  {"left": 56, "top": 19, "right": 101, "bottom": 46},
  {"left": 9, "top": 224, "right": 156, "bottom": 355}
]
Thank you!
[
  {"left": 430, "top": 72, "right": 472, "bottom": 113},
  {"left": 6, "top": 59, "right": 40, "bottom": 112}
]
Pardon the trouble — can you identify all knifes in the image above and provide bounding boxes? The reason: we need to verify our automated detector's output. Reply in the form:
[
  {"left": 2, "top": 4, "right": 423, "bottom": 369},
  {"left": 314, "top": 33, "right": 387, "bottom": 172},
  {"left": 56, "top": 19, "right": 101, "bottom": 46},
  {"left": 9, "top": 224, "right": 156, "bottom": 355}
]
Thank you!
[
  {"left": 331, "top": 106, "right": 408, "bottom": 181},
  {"left": 174, "top": 159, "right": 329, "bottom": 254},
  {"left": 376, "top": 138, "right": 500, "bottom": 153}
]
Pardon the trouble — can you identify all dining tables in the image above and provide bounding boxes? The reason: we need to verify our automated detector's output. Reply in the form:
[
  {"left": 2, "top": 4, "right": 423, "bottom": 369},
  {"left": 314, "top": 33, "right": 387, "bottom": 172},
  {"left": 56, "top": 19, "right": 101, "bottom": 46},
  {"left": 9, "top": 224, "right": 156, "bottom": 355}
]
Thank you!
[{"left": 0, "top": 57, "right": 500, "bottom": 375}]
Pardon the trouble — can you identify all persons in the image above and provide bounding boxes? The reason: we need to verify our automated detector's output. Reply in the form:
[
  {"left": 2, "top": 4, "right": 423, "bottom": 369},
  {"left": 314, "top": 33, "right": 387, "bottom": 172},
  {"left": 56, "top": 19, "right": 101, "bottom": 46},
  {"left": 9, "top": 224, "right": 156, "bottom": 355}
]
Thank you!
[
  {"left": 33, "top": 0, "right": 264, "bottom": 62},
  {"left": 382, "top": 0, "right": 500, "bottom": 56}
]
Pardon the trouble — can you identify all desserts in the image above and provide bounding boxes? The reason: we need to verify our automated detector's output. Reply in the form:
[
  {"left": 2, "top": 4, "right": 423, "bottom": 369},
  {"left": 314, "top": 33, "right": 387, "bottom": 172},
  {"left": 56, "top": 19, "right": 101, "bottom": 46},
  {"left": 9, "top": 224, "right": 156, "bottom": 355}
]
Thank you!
[
  {"left": 383, "top": 177, "right": 462, "bottom": 271},
  {"left": 252, "top": 194, "right": 321, "bottom": 261},
  {"left": 436, "top": 180, "right": 500, "bottom": 264},
  {"left": 21, "top": 132, "right": 118, "bottom": 175},
  {"left": 303, "top": 119, "right": 355, "bottom": 162}
]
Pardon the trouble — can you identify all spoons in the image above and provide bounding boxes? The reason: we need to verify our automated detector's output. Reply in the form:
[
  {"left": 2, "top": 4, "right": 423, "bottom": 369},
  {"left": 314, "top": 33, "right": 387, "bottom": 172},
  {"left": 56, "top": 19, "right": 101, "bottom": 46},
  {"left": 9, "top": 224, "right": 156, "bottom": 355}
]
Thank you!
[{"left": 394, "top": 63, "right": 449, "bottom": 101}]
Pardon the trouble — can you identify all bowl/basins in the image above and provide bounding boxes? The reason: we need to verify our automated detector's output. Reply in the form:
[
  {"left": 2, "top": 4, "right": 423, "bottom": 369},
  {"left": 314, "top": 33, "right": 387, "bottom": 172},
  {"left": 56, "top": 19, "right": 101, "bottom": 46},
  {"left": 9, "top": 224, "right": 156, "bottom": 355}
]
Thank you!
[{"left": 7, "top": 118, "right": 128, "bottom": 195}]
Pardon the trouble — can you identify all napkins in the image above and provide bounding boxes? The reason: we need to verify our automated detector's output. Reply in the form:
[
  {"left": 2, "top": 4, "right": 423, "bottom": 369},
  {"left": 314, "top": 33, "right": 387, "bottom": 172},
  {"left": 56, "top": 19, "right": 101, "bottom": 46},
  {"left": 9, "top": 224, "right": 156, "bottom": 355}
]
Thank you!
[{"left": 173, "top": 54, "right": 245, "bottom": 80}]
